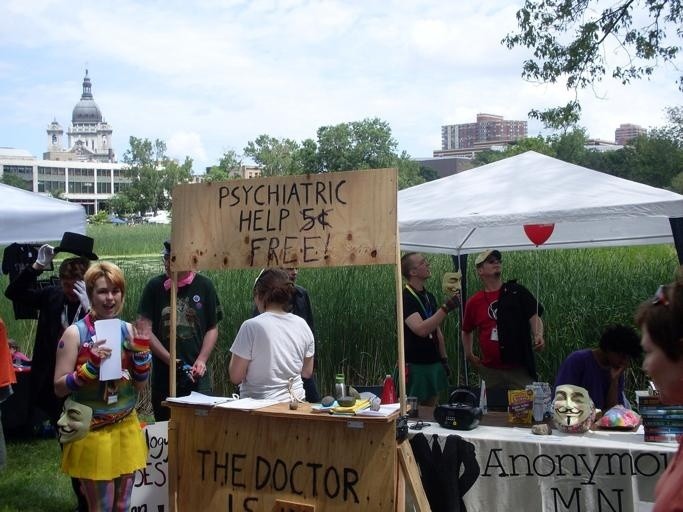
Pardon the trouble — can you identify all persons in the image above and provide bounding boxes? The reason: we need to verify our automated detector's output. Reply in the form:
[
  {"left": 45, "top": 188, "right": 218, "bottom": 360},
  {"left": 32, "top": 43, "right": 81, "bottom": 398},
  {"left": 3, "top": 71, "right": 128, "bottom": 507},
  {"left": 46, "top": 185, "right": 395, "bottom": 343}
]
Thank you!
[
  {"left": 52, "top": 261, "right": 153, "bottom": 512},
  {"left": 551, "top": 385, "right": 593, "bottom": 428},
  {"left": 401, "top": 251, "right": 462, "bottom": 405},
  {"left": 460, "top": 249, "right": 546, "bottom": 413},
  {"left": 56, "top": 397, "right": 94, "bottom": 445},
  {"left": 441, "top": 270, "right": 463, "bottom": 299},
  {"left": 1, "top": 319, "right": 29, "bottom": 468},
  {"left": 227, "top": 269, "right": 320, "bottom": 401},
  {"left": 136, "top": 240, "right": 223, "bottom": 422},
  {"left": 4, "top": 244, "right": 89, "bottom": 437},
  {"left": 631, "top": 276, "right": 682, "bottom": 512},
  {"left": 552, "top": 321, "right": 642, "bottom": 423}
]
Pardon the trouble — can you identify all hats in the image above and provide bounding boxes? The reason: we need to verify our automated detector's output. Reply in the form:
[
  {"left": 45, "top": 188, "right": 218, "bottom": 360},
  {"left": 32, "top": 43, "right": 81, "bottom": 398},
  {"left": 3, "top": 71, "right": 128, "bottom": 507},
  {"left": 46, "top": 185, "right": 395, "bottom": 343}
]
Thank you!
[
  {"left": 160, "top": 239, "right": 171, "bottom": 255},
  {"left": 54, "top": 232, "right": 98, "bottom": 260},
  {"left": 475, "top": 249, "right": 501, "bottom": 265}
]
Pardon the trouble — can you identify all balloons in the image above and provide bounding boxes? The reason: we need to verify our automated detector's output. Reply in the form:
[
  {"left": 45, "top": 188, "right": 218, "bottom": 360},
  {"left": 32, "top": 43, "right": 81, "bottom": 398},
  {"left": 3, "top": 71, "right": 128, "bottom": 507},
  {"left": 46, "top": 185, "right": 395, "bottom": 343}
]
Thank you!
[{"left": 523, "top": 222, "right": 555, "bottom": 248}]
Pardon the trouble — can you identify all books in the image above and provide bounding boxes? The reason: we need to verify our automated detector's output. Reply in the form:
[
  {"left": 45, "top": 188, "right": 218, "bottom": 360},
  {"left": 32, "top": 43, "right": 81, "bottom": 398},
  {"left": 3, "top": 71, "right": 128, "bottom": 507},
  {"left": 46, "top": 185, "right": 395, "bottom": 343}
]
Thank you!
[{"left": 634, "top": 380, "right": 683, "bottom": 446}]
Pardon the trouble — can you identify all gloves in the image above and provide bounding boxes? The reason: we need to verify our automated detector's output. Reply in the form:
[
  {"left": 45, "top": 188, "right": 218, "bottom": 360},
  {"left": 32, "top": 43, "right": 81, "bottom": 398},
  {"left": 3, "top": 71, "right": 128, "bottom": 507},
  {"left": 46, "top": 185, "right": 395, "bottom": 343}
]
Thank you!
[
  {"left": 441, "top": 357, "right": 451, "bottom": 376},
  {"left": 440, "top": 295, "right": 460, "bottom": 314},
  {"left": 72, "top": 280, "right": 91, "bottom": 313},
  {"left": 36, "top": 244, "right": 54, "bottom": 267},
  {"left": 174, "top": 360, "right": 196, "bottom": 384}
]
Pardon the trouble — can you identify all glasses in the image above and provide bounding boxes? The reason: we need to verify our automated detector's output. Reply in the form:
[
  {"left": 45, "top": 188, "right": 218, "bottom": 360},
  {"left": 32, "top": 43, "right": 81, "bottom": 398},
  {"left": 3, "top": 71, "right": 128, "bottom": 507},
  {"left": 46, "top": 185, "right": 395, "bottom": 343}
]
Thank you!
[
  {"left": 652, "top": 284, "right": 670, "bottom": 307},
  {"left": 486, "top": 256, "right": 501, "bottom": 264}
]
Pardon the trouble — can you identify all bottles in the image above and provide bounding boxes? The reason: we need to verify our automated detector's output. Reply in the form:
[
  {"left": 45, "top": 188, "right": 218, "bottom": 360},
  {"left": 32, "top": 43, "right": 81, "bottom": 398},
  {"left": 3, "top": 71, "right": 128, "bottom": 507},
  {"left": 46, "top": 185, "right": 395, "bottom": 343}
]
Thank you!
[
  {"left": 383, "top": 374, "right": 396, "bottom": 404},
  {"left": 406, "top": 396, "right": 418, "bottom": 419},
  {"left": 335, "top": 374, "right": 346, "bottom": 405},
  {"left": 525, "top": 381, "right": 551, "bottom": 422}
]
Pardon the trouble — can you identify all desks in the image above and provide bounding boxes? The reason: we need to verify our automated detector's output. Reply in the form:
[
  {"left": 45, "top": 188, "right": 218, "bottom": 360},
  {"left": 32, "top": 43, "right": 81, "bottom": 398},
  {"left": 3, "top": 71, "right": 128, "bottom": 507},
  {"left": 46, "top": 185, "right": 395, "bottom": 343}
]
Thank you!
[
  {"left": 161, "top": 391, "right": 412, "bottom": 511},
  {"left": 413, "top": 421, "right": 678, "bottom": 511}
]
